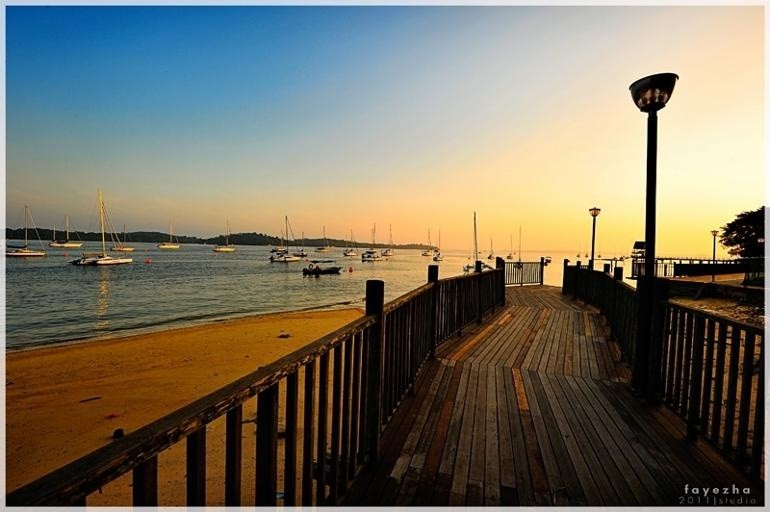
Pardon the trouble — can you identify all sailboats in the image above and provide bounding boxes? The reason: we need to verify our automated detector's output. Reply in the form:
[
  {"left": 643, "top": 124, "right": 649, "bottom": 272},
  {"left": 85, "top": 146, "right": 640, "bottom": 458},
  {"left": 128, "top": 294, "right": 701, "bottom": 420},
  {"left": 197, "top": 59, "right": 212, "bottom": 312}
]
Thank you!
[
  {"left": 344, "top": 224, "right": 393, "bottom": 261},
  {"left": 507, "top": 226, "right": 523, "bottom": 268},
  {"left": 6, "top": 206, "right": 47, "bottom": 257},
  {"left": 212, "top": 220, "right": 236, "bottom": 252},
  {"left": 421, "top": 228, "right": 443, "bottom": 261},
  {"left": 66, "top": 192, "right": 135, "bottom": 265},
  {"left": 49, "top": 215, "right": 83, "bottom": 248},
  {"left": 268, "top": 216, "right": 343, "bottom": 274},
  {"left": 462, "top": 212, "right": 494, "bottom": 272},
  {"left": 157, "top": 223, "right": 180, "bottom": 249}
]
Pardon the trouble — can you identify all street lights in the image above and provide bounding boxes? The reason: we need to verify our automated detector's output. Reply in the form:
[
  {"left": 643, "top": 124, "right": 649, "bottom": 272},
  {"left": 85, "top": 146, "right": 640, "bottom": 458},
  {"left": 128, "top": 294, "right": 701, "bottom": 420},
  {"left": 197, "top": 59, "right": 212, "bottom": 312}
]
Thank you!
[
  {"left": 589, "top": 208, "right": 600, "bottom": 260},
  {"left": 711, "top": 231, "right": 719, "bottom": 282},
  {"left": 629, "top": 72, "right": 678, "bottom": 275}
]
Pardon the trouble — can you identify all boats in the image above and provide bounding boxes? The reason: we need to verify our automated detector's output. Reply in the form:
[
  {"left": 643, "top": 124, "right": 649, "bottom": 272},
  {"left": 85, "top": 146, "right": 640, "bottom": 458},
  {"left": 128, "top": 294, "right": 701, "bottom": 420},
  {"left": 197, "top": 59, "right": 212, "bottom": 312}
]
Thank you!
[{"left": 545, "top": 256, "right": 551, "bottom": 263}]
